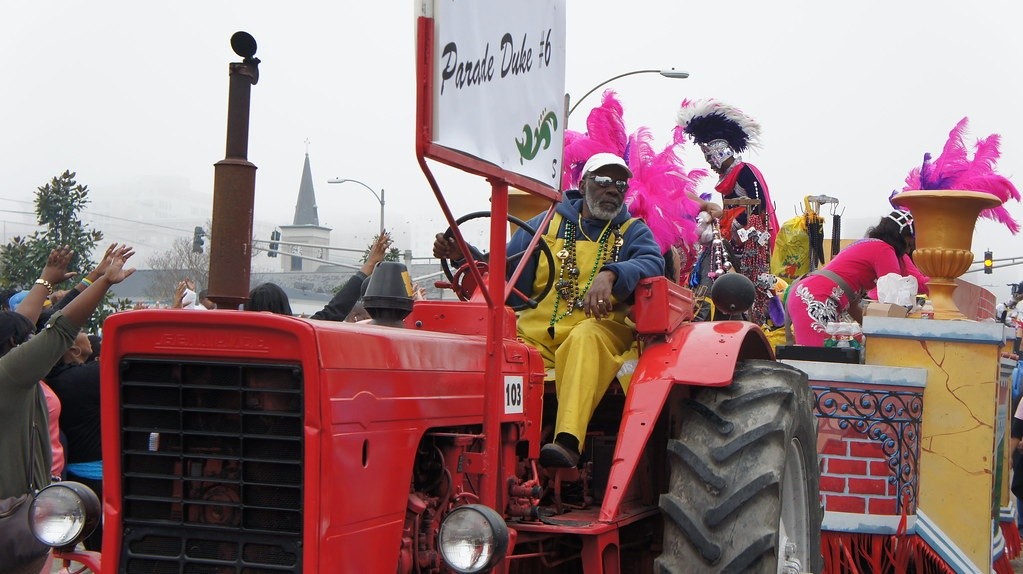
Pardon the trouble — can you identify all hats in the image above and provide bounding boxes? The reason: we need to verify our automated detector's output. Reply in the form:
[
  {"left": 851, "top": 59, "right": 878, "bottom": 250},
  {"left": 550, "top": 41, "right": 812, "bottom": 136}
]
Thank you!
[{"left": 581, "top": 153, "right": 633, "bottom": 184}]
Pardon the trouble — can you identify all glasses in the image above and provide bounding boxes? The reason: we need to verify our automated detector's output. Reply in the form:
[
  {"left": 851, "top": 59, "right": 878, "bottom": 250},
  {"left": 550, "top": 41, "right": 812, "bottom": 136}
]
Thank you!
[{"left": 587, "top": 175, "right": 629, "bottom": 193}]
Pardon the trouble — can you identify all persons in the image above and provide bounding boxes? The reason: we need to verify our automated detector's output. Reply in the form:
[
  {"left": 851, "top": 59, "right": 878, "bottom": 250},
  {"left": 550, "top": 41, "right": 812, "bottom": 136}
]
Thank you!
[
  {"left": 432, "top": 152, "right": 664, "bottom": 482},
  {"left": 244, "top": 229, "right": 427, "bottom": 322},
  {"left": 678, "top": 98, "right": 780, "bottom": 329},
  {"left": 787, "top": 209, "right": 931, "bottom": 348},
  {"left": 0, "top": 241, "right": 136, "bottom": 574},
  {"left": 995, "top": 281, "right": 1023, "bottom": 550},
  {"left": 133, "top": 276, "right": 216, "bottom": 311}
]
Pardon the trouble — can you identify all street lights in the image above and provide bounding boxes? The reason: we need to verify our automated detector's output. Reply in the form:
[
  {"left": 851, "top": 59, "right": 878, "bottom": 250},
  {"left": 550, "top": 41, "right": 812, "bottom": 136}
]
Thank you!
[
  {"left": 563, "top": 68, "right": 691, "bottom": 190},
  {"left": 326, "top": 177, "right": 386, "bottom": 239}
]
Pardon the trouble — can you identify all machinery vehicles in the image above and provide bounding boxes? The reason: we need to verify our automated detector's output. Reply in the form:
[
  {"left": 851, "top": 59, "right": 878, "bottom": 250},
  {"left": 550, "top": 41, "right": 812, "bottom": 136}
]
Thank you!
[{"left": 0, "top": 31, "right": 824, "bottom": 574}]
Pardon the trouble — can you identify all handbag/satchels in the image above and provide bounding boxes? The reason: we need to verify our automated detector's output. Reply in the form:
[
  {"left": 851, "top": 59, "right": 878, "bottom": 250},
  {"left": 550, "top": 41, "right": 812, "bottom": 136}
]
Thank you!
[
  {"left": 770, "top": 194, "right": 825, "bottom": 284},
  {"left": 0, "top": 493, "right": 49, "bottom": 572}
]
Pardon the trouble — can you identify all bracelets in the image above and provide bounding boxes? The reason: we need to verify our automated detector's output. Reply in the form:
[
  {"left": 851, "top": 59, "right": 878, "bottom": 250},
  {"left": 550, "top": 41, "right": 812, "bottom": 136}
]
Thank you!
[
  {"left": 81, "top": 280, "right": 90, "bottom": 288},
  {"left": 82, "top": 278, "right": 93, "bottom": 285},
  {"left": 34, "top": 279, "right": 53, "bottom": 294}
]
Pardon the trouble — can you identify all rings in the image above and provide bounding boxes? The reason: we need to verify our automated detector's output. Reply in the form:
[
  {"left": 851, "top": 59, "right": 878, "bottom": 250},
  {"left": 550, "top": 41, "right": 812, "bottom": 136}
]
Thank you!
[{"left": 596, "top": 299, "right": 603, "bottom": 305}]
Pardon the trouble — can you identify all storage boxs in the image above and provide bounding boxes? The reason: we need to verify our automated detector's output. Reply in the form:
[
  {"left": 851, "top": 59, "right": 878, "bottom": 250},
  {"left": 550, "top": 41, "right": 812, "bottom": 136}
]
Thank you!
[{"left": 867, "top": 301, "right": 909, "bottom": 318}]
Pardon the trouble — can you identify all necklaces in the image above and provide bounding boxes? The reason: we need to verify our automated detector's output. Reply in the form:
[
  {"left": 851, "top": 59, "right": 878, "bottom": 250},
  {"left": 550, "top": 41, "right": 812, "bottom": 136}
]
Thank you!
[{"left": 547, "top": 212, "right": 623, "bottom": 339}]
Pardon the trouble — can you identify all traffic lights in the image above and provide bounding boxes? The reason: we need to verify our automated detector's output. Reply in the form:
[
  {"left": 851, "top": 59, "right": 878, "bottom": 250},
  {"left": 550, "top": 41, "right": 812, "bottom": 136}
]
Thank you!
[{"left": 984, "top": 251, "right": 993, "bottom": 274}]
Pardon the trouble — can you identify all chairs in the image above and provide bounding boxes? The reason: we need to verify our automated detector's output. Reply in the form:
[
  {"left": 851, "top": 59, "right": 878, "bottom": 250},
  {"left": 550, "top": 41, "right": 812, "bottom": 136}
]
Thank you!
[{"left": 545, "top": 244, "right": 682, "bottom": 397}]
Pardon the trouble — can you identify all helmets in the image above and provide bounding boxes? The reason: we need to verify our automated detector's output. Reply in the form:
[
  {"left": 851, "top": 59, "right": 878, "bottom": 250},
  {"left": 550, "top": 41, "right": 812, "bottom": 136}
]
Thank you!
[{"left": 677, "top": 97, "right": 762, "bottom": 170}]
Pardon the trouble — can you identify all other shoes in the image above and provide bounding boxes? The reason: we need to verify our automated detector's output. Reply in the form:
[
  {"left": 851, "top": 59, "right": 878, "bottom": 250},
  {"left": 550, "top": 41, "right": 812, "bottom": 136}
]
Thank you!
[{"left": 539, "top": 438, "right": 585, "bottom": 507}]
